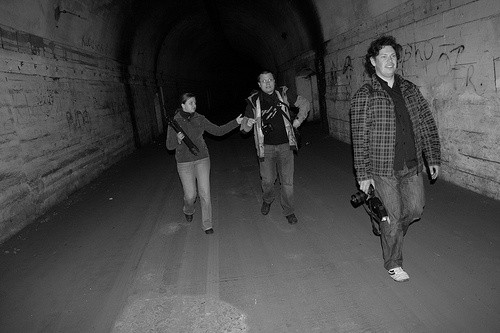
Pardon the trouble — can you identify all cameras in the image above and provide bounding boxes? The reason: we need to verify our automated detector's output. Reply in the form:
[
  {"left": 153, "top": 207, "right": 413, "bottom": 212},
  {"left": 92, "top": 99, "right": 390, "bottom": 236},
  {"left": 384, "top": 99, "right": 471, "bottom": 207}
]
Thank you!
[
  {"left": 351, "top": 184, "right": 388, "bottom": 221},
  {"left": 261, "top": 123, "right": 273, "bottom": 136}
]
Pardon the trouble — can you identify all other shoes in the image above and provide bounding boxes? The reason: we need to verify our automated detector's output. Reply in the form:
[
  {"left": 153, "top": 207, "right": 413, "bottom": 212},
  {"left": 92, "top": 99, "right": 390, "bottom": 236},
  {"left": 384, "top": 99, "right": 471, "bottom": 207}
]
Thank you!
[
  {"left": 287, "top": 213, "right": 297, "bottom": 224},
  {"left": 205, "top": 229, "right": 213, "bottom": 234},
  {"left": 261, "top": 202, "right": 270, "bottom": 214},
  {"left": 186, "top": 213, "right": 193, "bottom": 222}
]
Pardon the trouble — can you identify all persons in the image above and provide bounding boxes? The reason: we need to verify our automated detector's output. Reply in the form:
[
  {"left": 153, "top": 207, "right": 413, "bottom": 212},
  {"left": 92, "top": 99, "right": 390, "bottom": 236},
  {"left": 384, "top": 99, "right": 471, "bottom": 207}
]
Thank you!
[
  {"left": 165, "top": 91, "right": 244, "bottom": 235},
  {"left": 350, "top": 34, "right": 442, "bottom": 283},
  {"left": 240, "top": 70, "right": 312, "bottom": 225}
]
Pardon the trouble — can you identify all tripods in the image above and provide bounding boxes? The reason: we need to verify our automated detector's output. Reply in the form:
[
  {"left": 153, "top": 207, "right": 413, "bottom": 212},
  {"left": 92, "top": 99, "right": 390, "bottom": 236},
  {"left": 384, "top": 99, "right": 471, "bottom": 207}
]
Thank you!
[{"left": 167, "top": 115, "right": 199, "bottom": 154}]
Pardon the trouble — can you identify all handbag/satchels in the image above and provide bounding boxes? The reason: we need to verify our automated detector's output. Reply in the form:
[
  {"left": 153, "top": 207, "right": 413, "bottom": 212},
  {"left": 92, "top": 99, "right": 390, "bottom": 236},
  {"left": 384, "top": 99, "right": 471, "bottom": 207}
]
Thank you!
[{"left": 292, "top": 119, "right": 309, "bottom": 155}]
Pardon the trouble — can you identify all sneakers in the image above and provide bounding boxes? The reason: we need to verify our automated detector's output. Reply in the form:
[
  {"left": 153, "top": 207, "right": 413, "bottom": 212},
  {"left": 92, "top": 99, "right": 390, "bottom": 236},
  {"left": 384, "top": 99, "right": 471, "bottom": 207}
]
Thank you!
[{"left": 388, "top": 267, "right": 409, "bottom": 282}]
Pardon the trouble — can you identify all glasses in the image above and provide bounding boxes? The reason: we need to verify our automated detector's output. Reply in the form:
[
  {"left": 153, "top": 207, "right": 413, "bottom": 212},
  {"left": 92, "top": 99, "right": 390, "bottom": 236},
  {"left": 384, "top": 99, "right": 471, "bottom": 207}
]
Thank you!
[{"left": 260, "top": 78, "right": 274, "bottom": 84}]
